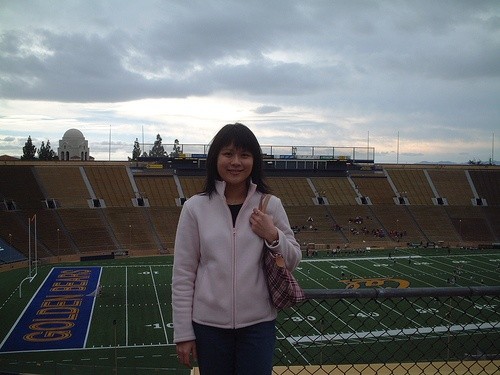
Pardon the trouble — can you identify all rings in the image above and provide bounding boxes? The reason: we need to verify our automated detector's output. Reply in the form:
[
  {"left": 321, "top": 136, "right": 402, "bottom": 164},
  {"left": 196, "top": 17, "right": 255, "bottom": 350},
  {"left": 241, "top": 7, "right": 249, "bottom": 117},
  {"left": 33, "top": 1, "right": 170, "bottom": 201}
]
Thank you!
[{"left": 256, "top": 210, "right": 260, "bottom": 215}]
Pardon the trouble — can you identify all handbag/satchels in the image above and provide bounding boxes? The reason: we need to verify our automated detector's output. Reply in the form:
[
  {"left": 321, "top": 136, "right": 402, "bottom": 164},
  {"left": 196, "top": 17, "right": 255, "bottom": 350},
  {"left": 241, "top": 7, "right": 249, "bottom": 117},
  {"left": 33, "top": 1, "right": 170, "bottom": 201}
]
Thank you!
[{"left": 259, "top": 194, "right": 306, "bottom": 309}]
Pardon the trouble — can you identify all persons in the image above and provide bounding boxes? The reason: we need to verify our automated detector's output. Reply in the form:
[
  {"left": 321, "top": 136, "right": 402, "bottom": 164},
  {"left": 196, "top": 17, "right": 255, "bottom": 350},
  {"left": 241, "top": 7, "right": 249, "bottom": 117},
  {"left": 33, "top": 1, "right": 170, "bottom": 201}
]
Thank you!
[
  {"left": 291, "top": 216, "right": 475, "bottom": 286},
  {"left": 172, "top": 124, "right": 302, "bottom": 375}
]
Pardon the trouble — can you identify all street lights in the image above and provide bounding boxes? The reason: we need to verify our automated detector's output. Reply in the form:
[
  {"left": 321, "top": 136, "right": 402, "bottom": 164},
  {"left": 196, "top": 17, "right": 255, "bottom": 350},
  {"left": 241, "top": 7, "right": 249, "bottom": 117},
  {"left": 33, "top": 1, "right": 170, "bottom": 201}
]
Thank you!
[
  {"left": 396, "top": 219, "right": 399, "bottom": 244},
  {"left": 9, "top": 234, "right": 13, "bottom": 266},
  {"left": 127, "top": 224, "right": 133, "bottom": 256},
  {"left": 459, "top": 220, "right": 462, "bottom": 243},
  {"left": 57, "top": 228, "right": 60, "bottom": 261}
]
